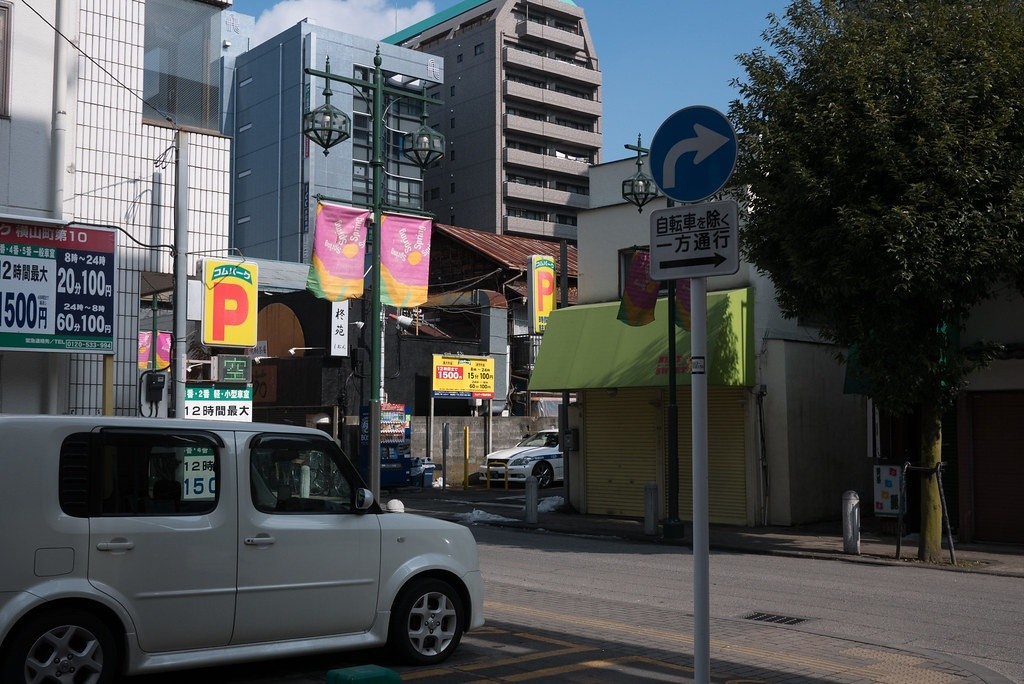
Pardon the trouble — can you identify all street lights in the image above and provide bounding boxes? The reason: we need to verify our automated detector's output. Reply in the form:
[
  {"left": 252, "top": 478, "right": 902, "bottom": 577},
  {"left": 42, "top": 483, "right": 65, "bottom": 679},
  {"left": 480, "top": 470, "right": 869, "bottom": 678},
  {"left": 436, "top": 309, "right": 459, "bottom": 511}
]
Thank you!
[
  {"left": 625, "top": 133, "right": 684, "bottom": 545},
  {"left": 302, "top": 44, "right": 447, "bottom": 512}
]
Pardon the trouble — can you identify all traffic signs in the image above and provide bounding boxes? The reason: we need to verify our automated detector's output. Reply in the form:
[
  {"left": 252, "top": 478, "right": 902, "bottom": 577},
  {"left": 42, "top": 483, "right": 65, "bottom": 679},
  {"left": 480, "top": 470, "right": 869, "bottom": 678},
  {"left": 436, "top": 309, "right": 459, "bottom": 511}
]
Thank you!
[{"left": 649, "top": 198, "right": 739, "bottom": 281}]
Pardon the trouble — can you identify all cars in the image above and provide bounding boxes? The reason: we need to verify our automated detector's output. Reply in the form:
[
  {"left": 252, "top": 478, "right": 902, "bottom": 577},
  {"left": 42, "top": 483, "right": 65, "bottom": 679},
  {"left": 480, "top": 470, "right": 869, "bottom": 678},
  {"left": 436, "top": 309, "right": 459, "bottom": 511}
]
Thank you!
[
  {"left": 478, "top": 428, "right": 563, "bottom": 489},
  {"left": 0, "top": 412, "right": 485, "bottom": 684}
]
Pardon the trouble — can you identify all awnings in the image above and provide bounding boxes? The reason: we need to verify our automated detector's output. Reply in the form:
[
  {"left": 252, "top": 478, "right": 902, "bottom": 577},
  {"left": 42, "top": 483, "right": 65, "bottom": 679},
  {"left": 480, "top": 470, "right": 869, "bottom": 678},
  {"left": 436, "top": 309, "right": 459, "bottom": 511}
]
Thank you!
[{"left": 527, "top": 286, "right": 756, "bottom": 391}]
